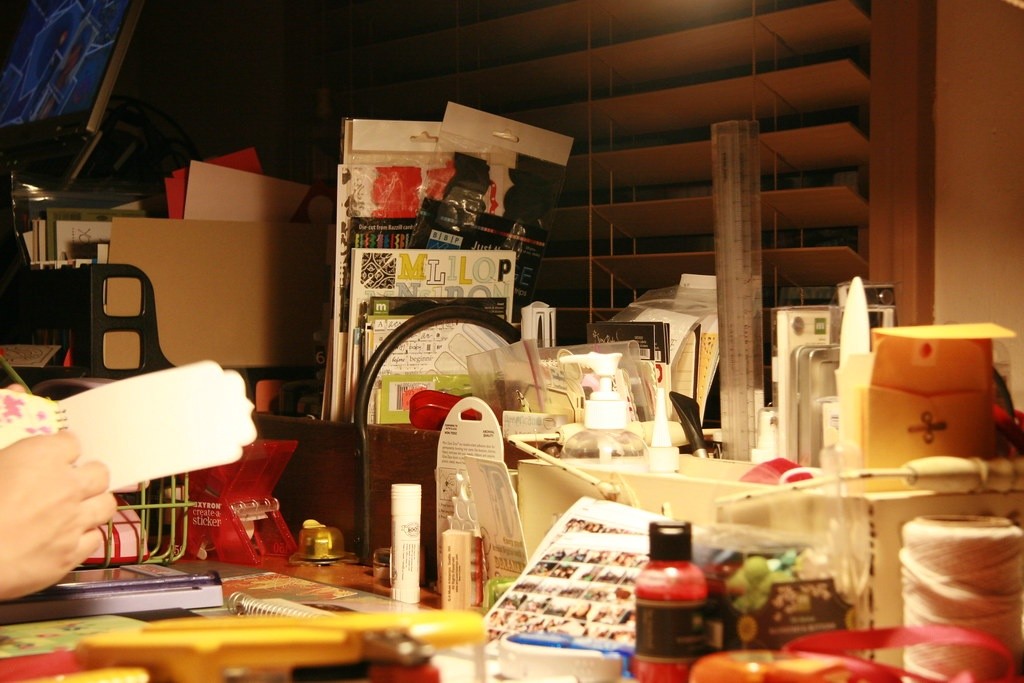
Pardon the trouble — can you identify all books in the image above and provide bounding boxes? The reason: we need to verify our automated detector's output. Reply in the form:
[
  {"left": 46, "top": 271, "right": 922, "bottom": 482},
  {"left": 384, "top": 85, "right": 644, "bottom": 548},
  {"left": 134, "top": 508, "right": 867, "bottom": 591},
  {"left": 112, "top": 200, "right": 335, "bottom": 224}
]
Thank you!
[
  {"left": 767, "top": 285, "right": 895, "bottom": 465},
  {"left": 344, "top": 196, "right": 548, "bottom": 426},
  {"left": 24, "top": 200, "right": 149, "bottom": 372},
  {"left": 588, "top": 304, "right": 701, "bottom": 424}
]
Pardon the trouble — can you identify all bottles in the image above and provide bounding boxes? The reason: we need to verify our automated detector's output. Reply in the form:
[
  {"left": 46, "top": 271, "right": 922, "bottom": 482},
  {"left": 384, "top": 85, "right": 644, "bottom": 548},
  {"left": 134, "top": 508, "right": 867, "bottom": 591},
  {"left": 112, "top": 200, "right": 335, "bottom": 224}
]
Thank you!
[{"left": 634, "top": 522, "right": 708, "bottom": 683}]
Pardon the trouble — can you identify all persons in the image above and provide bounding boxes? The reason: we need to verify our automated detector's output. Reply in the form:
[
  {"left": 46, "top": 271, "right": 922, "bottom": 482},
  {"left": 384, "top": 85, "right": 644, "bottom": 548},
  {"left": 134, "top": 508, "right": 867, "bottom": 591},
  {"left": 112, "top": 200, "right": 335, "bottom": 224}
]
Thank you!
[{"left": 0, "top": 426, "right": 119, "bottom": 602}]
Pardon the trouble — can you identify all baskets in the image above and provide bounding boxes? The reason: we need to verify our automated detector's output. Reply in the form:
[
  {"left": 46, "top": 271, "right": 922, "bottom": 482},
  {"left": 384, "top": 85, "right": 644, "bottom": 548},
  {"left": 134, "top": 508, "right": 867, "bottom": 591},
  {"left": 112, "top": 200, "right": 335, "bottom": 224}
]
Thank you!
[{"left": 77, "top": 473, "right": 189, "bottom": 567}]
